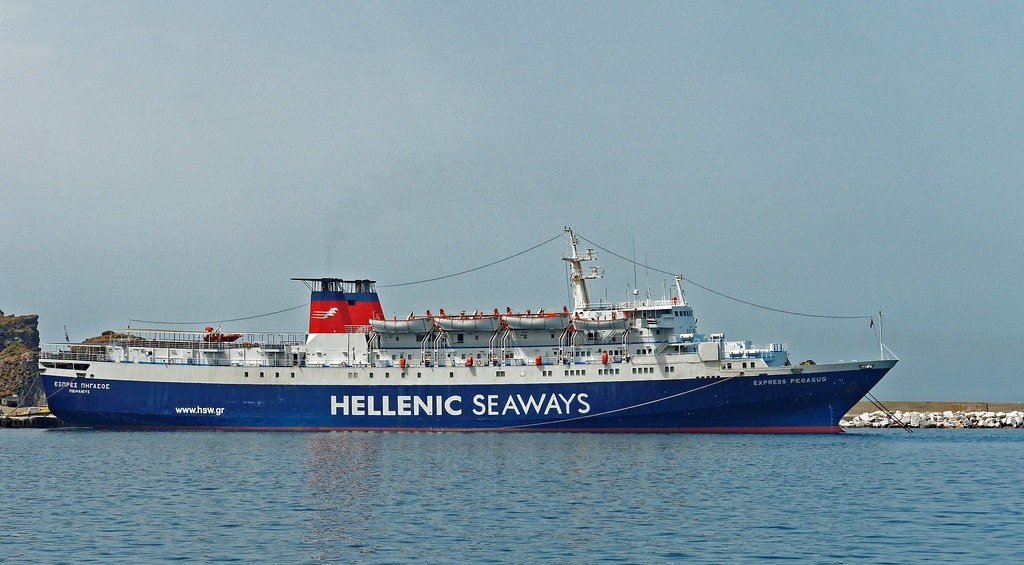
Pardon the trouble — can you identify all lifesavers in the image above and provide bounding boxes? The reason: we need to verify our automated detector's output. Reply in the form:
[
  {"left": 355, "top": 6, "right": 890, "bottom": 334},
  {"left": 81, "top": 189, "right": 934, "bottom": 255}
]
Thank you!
[{"left": 340, "top": 362, "right": 346, "bottom": 367}]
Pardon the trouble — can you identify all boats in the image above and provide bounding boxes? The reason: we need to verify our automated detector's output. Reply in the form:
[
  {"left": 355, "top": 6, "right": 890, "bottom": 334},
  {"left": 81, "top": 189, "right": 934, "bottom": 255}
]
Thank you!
[
  {"left": 203, "top": 333, "right": 242, "bottom": 343},
  {"left": 367, "top": 310, "right": 435, "bottom": 334},
  {"left": 572, "top": 311, "right": 632, "bottom": 330},
  {"left": 35, "top": 223, "right": 900, "bottom": 441},
  {"left": 500, "top": 304, "right": 572, "bottom": 329},
  {"left": 434, "top": 305, "right": 501, "bottom": 333}
]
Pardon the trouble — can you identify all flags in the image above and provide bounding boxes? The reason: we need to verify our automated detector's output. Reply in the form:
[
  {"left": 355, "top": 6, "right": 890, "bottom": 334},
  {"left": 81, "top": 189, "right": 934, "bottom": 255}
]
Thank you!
[{"left": 870, "top": 319, "right": 874, "bottom": 328}]
